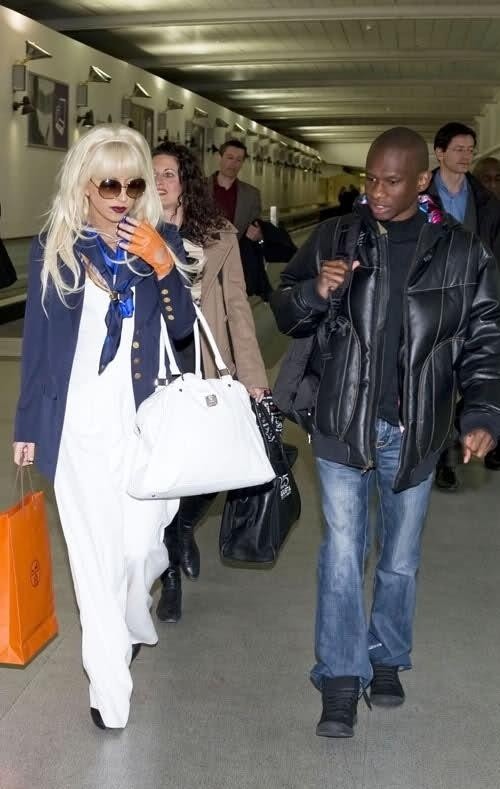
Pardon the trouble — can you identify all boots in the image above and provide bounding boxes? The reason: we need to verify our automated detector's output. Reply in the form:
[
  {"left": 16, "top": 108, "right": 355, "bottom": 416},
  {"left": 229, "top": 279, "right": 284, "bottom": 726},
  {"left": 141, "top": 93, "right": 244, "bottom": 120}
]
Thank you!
[
  {"left": 156, "top": 512, "right": 182, "bottom": 624},
  {"left": 176, "top": 491, "right": 219, "bottom": 581}
]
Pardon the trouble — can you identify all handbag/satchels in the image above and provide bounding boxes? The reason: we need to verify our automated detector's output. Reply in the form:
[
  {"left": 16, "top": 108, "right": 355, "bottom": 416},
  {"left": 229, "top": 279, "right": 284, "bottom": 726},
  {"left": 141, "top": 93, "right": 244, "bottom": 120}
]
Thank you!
[
  {"left": 0, "top": 488, "right": 62, "bottom": 667},
  {"left": 124, "top": 303, "right": 280, "bottom": 500},
  {"left": 219, "top": 402, "right": 301, "bottom": 565}
]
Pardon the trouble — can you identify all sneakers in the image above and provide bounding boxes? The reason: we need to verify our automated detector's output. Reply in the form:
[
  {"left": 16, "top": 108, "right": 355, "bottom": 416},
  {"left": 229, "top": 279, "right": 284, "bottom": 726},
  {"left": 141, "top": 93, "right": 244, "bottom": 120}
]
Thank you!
[
  {"left": 484, "top": 446, "right": 500, "bottom": 471},
  {"left": 435, "top": 464, "right": 459, "bottom": 491},
  {"left": 369, "top": 663, "right": 405, "bottom": 707},
  {"left": 315, "top": 675, "right": 361, "bottom": 739}
]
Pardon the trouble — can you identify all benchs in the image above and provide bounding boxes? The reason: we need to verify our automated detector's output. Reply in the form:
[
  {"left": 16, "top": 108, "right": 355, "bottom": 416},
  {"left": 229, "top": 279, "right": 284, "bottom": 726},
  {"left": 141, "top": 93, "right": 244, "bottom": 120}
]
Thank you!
[{"left": 0, "top": 235, "right": 38, "bottom": 307}]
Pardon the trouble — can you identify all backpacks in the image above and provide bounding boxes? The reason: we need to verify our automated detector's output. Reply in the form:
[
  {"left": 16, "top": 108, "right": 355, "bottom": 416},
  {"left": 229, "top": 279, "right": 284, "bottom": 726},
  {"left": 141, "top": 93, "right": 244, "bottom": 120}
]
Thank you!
[{"left": 271, "top": 215, "right": 365, "bottom": 432}]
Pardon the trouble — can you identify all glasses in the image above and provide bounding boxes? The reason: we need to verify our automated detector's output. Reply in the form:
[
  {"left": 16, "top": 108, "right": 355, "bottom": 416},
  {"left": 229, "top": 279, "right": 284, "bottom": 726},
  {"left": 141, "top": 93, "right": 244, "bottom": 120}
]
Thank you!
[
  {"left": 90, "top": 177, "right": 146, "bottom": 200},
  {"left": 449, "top": 146, "right": 475, "bottom": 155}
]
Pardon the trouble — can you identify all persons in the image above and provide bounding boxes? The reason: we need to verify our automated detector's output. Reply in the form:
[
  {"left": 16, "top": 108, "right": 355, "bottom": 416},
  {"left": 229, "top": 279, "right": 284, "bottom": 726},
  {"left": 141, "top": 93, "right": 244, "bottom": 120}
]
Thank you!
[
  {"left": 150, "top": 141, "right": 270, "bottom": 623},
  {"left": 10, "top": 121, "right": 198, "bottom": 730},
  {"left": 419, "top": 123, "right": 498, "bottom": 492},
  {"left": 205, "top": 138, "right": 265, "bottom": 248},
  {"left": 270, "top": 128, "right": 499, "bottom": 739}
]
